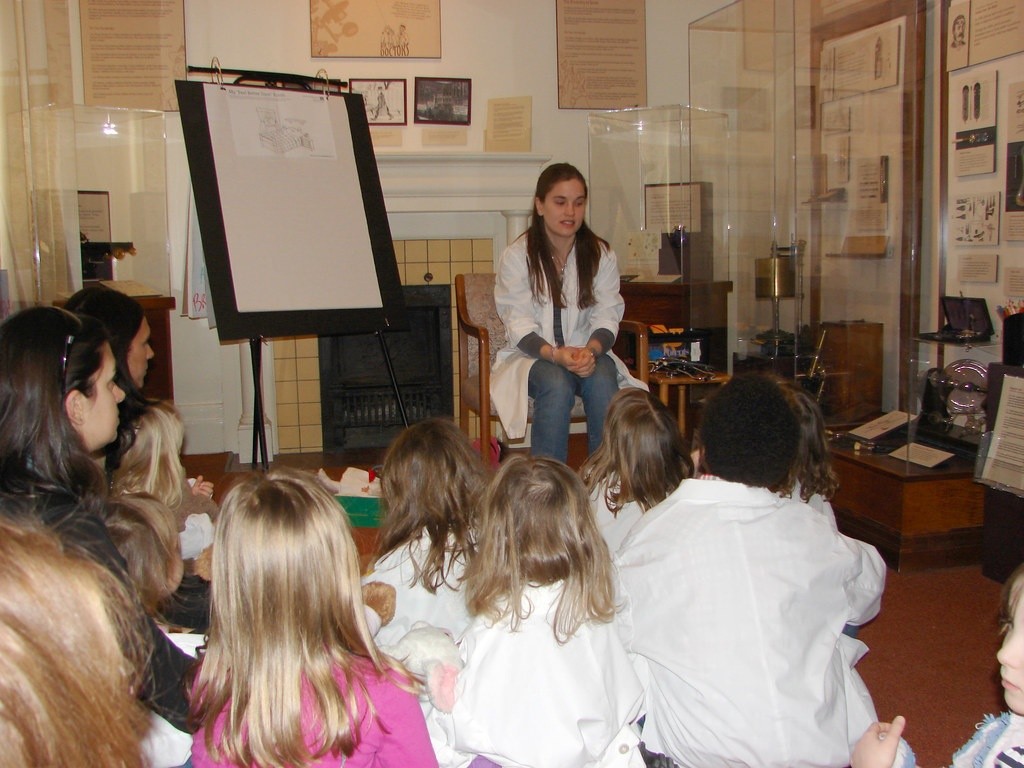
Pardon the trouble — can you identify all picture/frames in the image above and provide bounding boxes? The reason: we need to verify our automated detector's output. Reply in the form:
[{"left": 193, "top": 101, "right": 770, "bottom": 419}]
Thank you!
[
  {"left": 414, "top": 77, "right": 471, "bottom": 126},
  {"left": 349, "top": 79, "right": 407, "bottom": 126},
  {"left": 310, "top": 0, "right": 441, "bottom": 58},
  {"left": 78, "top": 190, "right": 113, "bottom": 280}
]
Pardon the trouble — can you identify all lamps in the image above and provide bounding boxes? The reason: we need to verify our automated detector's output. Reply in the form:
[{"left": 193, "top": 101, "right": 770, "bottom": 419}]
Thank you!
[{"left": 755, "top": 239, "right": 795, "bottom": 341}]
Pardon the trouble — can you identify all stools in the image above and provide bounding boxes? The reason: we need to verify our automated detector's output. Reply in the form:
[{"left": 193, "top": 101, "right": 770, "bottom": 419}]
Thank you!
[{"left": 648, "top": 361, "right": 738, "bottom": 439}]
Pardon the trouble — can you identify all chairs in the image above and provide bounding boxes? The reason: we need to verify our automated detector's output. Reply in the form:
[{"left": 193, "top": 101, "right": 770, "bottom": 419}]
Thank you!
[{"left": 454, "top": 272, "right": 648, "bottom": 470}]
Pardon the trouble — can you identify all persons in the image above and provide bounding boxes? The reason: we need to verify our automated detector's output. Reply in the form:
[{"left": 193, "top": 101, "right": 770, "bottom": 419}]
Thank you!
[
  {"left": 489, "top": 163, "right": 650, "bottom": 465},
  {"left": 950, "top": 14, "right": 967, "bottom": 48},
  {"left": 0, "top": 284, "right": 1023, "bottom": 766}
]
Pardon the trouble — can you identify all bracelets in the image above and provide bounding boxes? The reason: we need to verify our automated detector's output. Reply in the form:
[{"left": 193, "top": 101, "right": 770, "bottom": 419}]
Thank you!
[{"left": 551, "top": 347, "right": 556, "bottom": 364}]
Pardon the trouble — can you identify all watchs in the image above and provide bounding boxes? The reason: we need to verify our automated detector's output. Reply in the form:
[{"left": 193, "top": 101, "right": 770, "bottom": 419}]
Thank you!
[{"left": 586, "top": 346, "right": 599, "bottom": 359}]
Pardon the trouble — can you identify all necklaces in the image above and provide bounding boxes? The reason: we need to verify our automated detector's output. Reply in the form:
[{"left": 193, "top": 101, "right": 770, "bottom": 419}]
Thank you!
[{"left": 551, "top": 251, "right": 571, "bottom": 281}]
